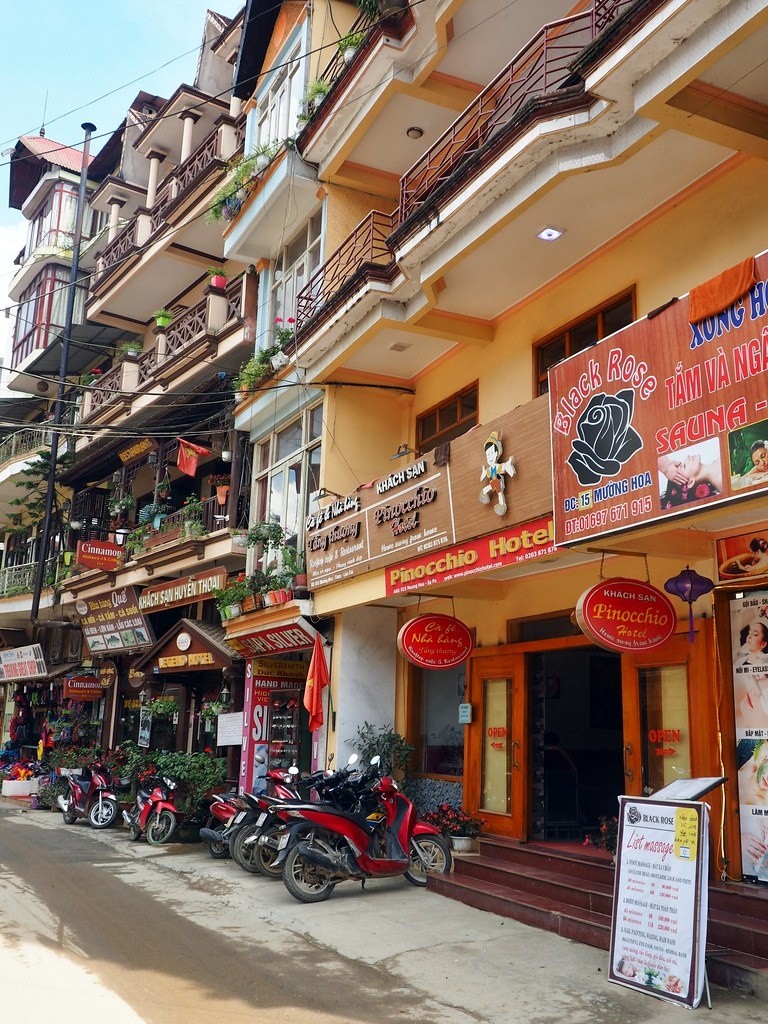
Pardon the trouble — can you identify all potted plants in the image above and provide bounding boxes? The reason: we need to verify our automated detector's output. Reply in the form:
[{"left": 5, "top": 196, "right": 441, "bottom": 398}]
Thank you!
[
  {"left": 116, "top": 793, "right": 135, "bottom": 811},
  {"left": 205, "top": 266, "right": 228, "bottom": 288},
  {"left": 157, "top": 483, "right": 170, "bottom": 498},
  {"left": 269, "top": 351, "right": 288, "bottom": 370},
  {"left": 299, "top": 78, "right": 333, "bottom": 108},
  {"left": 338, "top": 32, "right": 366, "bottom": 63},
  {"left": 178, "top": 518, "right": 208, "bottom": 544},
  {"left": 111, "top": 764, "right": 132, "bottom": 785},
  {"left": 281, "top": 545, "right": 307, "bottom": 586},
  {"left": 144, "top": 503, "right": 176, "bottom": 531},
  {"left": 64, "top": 548, "right": 76, "bottom": 566},
  {"left": 108, "top": 495, "right": 135, "bottom": 516},
  {"left": 153, "top": 306, "right": 173, "bottom": 327},
  {"left": 119, "top": 340, "right": 143, "bottom": 356},
  {"left": 296, "top": 114, "right": 312, "bottom": 131},
  {"left": 231, "top": 345, "right": 280, "bottom": 403},
  {"left": 204, "top": 142, "right": 274, "bottom": 225}
]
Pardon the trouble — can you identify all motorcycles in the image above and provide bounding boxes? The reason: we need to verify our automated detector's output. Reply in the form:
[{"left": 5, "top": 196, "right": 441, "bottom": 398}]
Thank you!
[
  {"left": 57, "top": 750, "right": 118, "bottom": 829},
  {"left": 200, "top": 752, "right": 451, "bottom": 903},
  {"left": 121, "top": 765, "right": 182, "bottom": 844}
]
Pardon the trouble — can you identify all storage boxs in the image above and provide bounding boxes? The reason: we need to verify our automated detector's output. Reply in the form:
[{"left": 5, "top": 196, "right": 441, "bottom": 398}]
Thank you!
[{"left": 2, "top": 778, "right": 38, "bottom": 797}]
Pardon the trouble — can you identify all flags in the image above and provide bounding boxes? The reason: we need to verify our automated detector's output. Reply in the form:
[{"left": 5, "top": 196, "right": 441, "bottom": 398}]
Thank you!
[
  {"left": 303, "top": 632, "right": 329, "bottom": 732},
  {"left": 177, "top": 442, "right": 211, "bottom": 477}
]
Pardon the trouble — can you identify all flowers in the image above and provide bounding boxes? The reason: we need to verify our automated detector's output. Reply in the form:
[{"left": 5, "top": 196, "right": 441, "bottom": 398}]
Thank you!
[
  {"left": 142, "top": 697, "right": 180, "bottom": 722},
  {"left": 197, "top": 698, "right": 232, "bottom": 720},
  {"left": 582, "top": 815, "right": 618, "bottom": 855},
  {"left": 274, "top": 317, "right": 295, "bottom": 346},
  {"left": 83, "top": 369, "right": 103, "bottom": 385},
  {"left": 231, "top": 521, "right": 288, "bottom": 553},
  {"left": 56, "top": 746, "right": 91, "bottom": 768},
  {"left": 137, "top": 747, "right": 226, "bottom": 824},
  {"left": 207, "top": 474, "right": 232, "bottom": 486},
  {"left": 110, "top": 518, "right": 134, "bottom": 531},
  {"left": 420, "top": 803, "right": 487, "bottom": 839},
  {"left": 210, "top": 560, "right": 289, "bottom": 609}
]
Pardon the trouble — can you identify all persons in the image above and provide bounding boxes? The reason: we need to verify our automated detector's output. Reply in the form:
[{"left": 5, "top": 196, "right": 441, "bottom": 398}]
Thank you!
[
  {"left": 738, "top": 755, "right": 768, "bottom": 806},
  {"left": 731, "top": 607, "right": 768, "bottom": 666},
  {"left": 736, "top": 537, "right": 768, "bottom": 571},
  {"left": 659, "top": 454, "right": 722, "bottom": 507},
  {"left": 742, "top": 440, "right": 768, "bottom": 480},
  {"left": 741, "top": 815, "right": 768, "bottom": 875},
  {"left": 735, "top": 674, "right": 768, "bottom": 728}
]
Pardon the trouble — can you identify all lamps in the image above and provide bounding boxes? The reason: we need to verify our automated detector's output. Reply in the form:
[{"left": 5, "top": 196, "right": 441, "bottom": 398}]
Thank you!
[
  {"left": 536, "top": 224, "right": 565, "bottom": 241},
  {"left": 407, "top": 127, "right": 423, "bottom": 139},
  {"left": 112, "top": 468, "right": 122, "bottom": 489},
  {"left": 13, "top": 513, "right": 22, "bottom": 526},
  {"left": 313, "top": 487, "right": 344, "bottom": 501},
  {"left": 63, "top": 498, "right": 70, "bottom": 514},
  {"left": 148, "top": 450, "right": 158, "bottom": 468},
  {"left": 390, "top": 444, "right": 424, "bottom": 460}
]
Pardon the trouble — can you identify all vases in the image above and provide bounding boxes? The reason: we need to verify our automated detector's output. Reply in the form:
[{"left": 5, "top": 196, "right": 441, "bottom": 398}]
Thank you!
[
  {"left": 157, "top": 708, "right": 163, "bottom": 714},
  {"left": 216, "top": 486, "right": 229, "bottom": 505},
  {"left": 115, "top": 528, "right": 130, "bottom": 545},
  {"left": 233, "top": 535, "right": 248, "bottom": 547},
  {"left": 260, "top": 525, "right": 274, "bottom": 538},
  {"left": 89, "top": 379, "right": 98, "bottom": 386},
  {"left": 217, "top": 589, "right": 291, "bottom": 621},
  {"left": 60, "top": 768, "right": 82, "bottom": 776},
  {"left": 166, "top": 825, "right": 205, "bottom": 843},
  {"left": 613, "top": 854, "right": 616, "bottom": 864},
  {"left": 212, "top": 707, "right": 220, "bottom": 715},
  {"left": 450, "top": 836, "right": 472, "bottom": 853},
  {"left": 148, "top": 763, "right": 157, "bottom": 771},
  {"left": 439, "top": 834, "right": 444, "bottom": 839}
]
